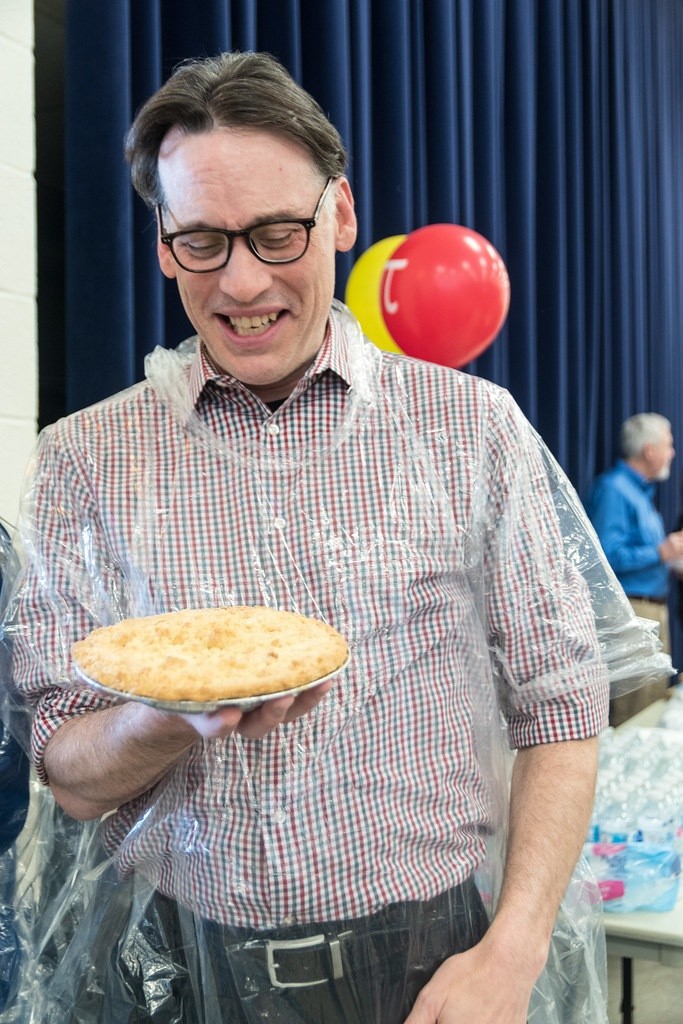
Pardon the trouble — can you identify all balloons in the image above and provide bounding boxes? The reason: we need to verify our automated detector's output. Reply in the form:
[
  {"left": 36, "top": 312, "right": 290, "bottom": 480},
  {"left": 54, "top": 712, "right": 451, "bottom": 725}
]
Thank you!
[{"left": 345, "top": 223, "right": 510, "bottom": 369}]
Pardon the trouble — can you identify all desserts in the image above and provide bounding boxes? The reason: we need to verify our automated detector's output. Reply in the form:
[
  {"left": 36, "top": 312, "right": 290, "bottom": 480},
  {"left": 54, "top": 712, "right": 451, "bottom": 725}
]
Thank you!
[{"left": 71, "top": 605, "right": 351, "bottom": 714}]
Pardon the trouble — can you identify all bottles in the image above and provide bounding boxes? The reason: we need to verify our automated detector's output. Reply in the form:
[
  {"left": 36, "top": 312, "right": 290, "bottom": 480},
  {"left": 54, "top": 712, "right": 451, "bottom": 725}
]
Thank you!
[{"left": 585, "top": 725, "right": 683, "bottom": 910}]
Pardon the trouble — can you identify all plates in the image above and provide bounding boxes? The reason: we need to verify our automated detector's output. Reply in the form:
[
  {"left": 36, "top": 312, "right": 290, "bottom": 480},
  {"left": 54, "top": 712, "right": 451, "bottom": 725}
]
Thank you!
[{"left": 74, "top": 616, "right": 351, "bottom": 714}]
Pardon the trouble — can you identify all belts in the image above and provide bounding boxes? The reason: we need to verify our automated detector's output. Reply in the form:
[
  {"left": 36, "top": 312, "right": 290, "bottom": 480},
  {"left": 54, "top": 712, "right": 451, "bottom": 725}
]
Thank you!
[
  {"left": 173, "top": 884, "right": 483, "bottom": 994},
  {"left": 626, "top": 595, "right": 669, "bottom": 605}
]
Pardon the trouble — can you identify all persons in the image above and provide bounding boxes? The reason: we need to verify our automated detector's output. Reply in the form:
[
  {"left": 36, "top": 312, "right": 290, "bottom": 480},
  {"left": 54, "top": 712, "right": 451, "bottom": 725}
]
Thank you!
[
  {"left": 0, "top": 49, "right": 677, "bottom": 1024},
  {"left": 591, "top": 413, "right": 683, "bottom": 727}
]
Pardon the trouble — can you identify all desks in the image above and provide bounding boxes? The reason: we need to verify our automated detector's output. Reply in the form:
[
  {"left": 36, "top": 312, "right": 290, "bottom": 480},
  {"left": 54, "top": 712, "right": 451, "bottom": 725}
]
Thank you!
[{"left": 555, "top": 678, "right": 680, "bottom": 1024}]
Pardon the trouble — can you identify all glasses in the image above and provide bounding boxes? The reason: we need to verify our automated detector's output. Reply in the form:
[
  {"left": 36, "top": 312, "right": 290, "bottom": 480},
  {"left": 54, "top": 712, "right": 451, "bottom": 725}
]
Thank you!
[{"left": 157, "top": 173, "right": 334, "bottom": 274}]
